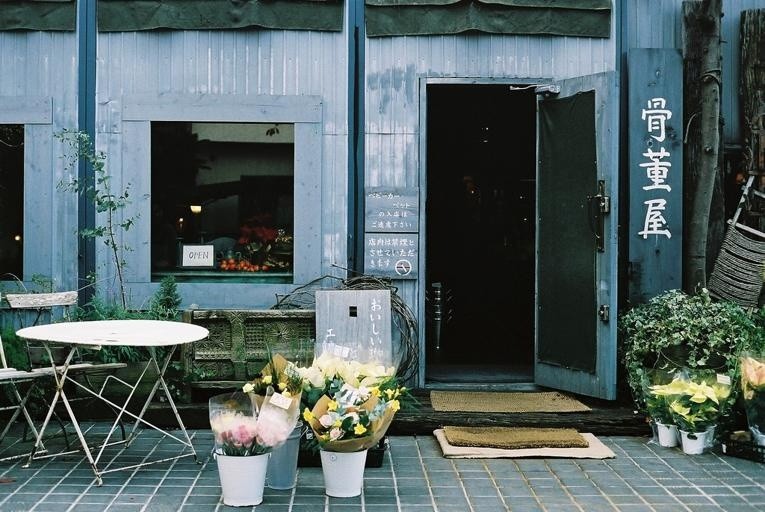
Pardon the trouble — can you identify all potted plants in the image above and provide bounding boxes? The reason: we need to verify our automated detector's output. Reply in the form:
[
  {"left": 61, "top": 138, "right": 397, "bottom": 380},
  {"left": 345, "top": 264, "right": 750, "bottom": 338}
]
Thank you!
[{"left": 26, "top": 273, "right": 183, "bottom": 397}]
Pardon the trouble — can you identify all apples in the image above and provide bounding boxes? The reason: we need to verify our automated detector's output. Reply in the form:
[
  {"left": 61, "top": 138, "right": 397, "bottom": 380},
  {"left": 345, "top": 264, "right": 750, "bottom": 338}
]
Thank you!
[{"left": 221, "top": 258, "right": 270, "bottom": 272}]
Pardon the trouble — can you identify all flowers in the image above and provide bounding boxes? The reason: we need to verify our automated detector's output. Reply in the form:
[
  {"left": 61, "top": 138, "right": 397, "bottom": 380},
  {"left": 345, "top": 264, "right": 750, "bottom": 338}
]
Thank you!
[
  {"left": 209, "top": 351, "right": 419, "bottom": 456},
  {"left": 646, "top": 357, "right": 765, "bottom": 432}
]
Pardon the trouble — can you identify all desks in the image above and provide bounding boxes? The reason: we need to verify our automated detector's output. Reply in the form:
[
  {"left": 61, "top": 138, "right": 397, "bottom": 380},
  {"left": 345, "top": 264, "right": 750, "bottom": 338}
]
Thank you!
[{"left": 15, "top": 320, "right": 209, "bottom": 485}]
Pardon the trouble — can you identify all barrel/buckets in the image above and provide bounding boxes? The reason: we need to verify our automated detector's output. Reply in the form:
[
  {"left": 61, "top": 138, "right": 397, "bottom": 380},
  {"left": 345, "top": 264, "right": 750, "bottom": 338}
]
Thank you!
[
  {"left": 319, "top": 450, "right": 368, "bottom": 498},
  {"left": 267, "top": 421, "right": 308, "bottom": 489},
  {"left": 215, "top": 452, "right": 269, "bottom": 507}
]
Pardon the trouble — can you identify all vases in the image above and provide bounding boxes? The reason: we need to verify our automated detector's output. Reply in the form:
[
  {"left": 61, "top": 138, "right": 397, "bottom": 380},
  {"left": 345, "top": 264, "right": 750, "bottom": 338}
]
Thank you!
[
  {"left": 758, "top": 435, "right": 765, "bottom": 453},
  {"left": 656, "top": 423, "right": 717, "bottom": 455},
  {"left": 214, "top": 421, "right": 367, "bottom": 506}
]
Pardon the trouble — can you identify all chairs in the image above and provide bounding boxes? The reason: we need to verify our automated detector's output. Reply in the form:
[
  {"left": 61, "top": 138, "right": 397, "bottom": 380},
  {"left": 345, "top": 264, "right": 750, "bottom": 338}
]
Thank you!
[
  {"left": 7, "top": 291, "right": 127, "bottom": 452},
  {"left": 0, "top": 336, "right": 48, "bottom": 461}
]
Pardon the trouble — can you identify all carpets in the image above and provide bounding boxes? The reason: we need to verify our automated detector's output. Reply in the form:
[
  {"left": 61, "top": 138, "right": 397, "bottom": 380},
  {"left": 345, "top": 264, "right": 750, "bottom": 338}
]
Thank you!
[{"left": 430, "top": 390, "right": 616, "bottom": 458}]
